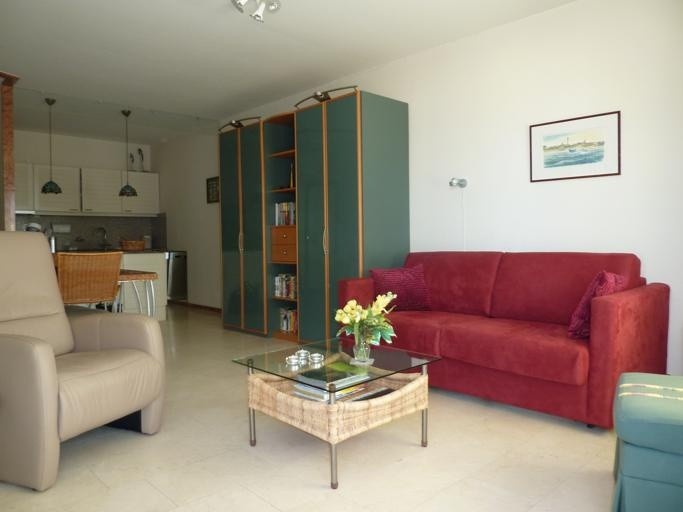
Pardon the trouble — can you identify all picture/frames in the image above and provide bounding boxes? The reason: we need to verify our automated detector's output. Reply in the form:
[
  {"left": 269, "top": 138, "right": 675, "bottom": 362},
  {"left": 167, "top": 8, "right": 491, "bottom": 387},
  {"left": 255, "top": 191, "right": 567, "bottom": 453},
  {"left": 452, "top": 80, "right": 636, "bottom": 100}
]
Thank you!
[
  {"left": 207, "top": 176, "right": 219, "bottom": 203},
  {"left": 530, "top": 111, "right": 620, "bottom": 182}
]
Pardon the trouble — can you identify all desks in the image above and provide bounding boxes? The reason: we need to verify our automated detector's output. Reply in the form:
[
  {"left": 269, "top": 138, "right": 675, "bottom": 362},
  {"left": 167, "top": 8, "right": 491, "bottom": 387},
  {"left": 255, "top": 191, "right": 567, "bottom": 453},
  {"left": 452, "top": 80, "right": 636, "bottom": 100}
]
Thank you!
[{"left": 118, "top": 269, "right": 158, "bottom": 317}]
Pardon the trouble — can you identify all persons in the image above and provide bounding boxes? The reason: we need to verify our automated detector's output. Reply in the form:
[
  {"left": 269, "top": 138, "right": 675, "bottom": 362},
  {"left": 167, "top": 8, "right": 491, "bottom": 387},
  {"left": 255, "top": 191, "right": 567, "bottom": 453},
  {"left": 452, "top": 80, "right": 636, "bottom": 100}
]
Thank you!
[{"left": 94, "top": 226, "right": 122, "bottom": 312}]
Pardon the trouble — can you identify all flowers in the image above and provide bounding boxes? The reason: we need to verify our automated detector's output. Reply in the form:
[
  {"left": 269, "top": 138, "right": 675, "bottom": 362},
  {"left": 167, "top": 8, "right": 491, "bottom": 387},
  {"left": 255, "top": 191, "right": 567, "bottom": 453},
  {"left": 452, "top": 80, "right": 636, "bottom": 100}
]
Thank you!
[{"left": 335, "top": 292, "right": 399, "bottom": 346}]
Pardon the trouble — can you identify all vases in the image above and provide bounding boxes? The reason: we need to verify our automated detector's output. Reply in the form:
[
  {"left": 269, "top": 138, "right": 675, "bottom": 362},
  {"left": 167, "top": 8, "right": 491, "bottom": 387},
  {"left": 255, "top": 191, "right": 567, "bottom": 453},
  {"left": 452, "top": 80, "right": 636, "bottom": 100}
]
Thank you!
[{"left": 352, "top": 342, "right": 371, "bottom": 362}]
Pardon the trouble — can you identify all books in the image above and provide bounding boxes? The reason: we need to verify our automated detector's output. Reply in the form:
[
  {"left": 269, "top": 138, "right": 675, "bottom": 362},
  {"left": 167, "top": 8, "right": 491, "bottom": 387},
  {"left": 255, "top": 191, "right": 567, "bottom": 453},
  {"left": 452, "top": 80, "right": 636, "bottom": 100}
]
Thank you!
[
  {"left": 280, "top": 308, "right": 297, "bottom": 335},
  {"left": 275, "top": 202, "right": 296, "bottom": 226},
  {"left": 292, "top": 361, "right": 387, "bottom": 404},
  {"left": 274, "top": 272, "right": 296, "bottom": 299}
]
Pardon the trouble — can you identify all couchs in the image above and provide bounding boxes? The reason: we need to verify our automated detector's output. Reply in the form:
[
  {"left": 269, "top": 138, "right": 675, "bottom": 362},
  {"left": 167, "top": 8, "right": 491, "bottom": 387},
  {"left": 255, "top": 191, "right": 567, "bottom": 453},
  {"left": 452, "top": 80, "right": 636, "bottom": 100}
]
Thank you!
[
  {"left": 338, "top": 252, "right": 670, "bottom": 429},
  {"left": 1, "top": 231, "right": 165, "bottom": 491},
  {"left": 613, "top": 373, "right": 682, "bottom": 511}
]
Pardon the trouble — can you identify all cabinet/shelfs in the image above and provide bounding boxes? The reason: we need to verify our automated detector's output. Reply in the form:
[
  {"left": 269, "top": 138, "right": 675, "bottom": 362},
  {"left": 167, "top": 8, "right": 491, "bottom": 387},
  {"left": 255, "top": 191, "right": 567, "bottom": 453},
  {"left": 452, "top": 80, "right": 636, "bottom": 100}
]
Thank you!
[
  {"left": 13, "top": 163, "right": 81, "bottom": 211},
  {"left": 14, "top": 212, "right": 169, "bottom": 308},
  {"left": 295, "top": 87, "right": 409, "bottom": 350},
  {"left": 219, "top": 116, "right": 267, "bottom": 337},
  {"left": 82, "top": 168, "right": 160, "bottom": 211},
  {"left": 260, "top": 113, "right": 298, "bottom": 341}
]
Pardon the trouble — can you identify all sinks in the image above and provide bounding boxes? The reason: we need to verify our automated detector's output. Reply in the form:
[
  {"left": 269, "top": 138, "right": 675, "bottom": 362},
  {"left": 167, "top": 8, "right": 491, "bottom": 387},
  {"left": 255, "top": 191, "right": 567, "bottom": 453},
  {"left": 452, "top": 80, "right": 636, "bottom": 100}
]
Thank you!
[{"left": 70, "top": 248, "right": 121, "bottom": 253}]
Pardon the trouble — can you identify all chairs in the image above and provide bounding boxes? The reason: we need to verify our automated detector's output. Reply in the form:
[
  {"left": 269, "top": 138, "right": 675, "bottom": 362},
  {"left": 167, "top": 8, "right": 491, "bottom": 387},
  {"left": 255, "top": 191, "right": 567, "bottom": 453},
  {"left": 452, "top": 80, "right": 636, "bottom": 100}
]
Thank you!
[{"left": 58, "top": 252, "right": 123, "bottom": 313}]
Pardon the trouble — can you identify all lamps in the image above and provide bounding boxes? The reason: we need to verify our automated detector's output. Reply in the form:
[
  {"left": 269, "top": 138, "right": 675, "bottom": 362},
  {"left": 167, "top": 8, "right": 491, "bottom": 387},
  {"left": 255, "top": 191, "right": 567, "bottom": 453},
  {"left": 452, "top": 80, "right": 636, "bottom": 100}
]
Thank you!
[
  {"left": 42, "top": 99, "right": 62, "bottom": 194},
  {"left": 119, "top": 110, "right": 138, "bottom": 196},
  {"left": 231, "top": 0, "right": 280, "bottom": 23}
]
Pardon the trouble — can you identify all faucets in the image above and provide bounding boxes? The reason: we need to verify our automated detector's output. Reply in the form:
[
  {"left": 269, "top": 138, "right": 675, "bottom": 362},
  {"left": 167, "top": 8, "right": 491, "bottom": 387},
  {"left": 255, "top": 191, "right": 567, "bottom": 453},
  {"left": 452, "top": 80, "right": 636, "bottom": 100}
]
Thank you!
[{"left": 97, "top": 227, "right": 108, "bottom": 250}]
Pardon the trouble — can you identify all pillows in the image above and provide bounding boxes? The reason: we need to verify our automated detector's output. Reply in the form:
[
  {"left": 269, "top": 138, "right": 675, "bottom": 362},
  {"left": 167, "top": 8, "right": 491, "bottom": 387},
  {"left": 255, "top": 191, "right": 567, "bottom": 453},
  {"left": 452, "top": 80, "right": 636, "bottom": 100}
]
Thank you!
[
  {"left": 369, "top": 264, "right": 432, "bottom": 312},
  {"left": 568, "top": 270, "right": 628, "bottom": 339}
]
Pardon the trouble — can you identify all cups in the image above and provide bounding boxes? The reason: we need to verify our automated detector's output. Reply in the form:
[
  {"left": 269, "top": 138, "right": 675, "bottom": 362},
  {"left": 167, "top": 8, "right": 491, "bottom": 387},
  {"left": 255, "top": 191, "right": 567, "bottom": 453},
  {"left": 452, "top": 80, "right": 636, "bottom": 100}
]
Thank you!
[
  {"left": 309, "top": 353, "right": 324, "bottom": 363},
  {"left": 295, "top": 349, "right": 311, "bottom": 359},
  {"left": 298, "top": 360, "right": 309, "bottom": 368},
  {"left": 286, "top": 364, "right": 299, "bottom": 372},
  {"left": 286, "top": 355, "right": 298, "bottom": 365},
  {"left": 308, "top": 362, "right": 323, "bottom": 369}
]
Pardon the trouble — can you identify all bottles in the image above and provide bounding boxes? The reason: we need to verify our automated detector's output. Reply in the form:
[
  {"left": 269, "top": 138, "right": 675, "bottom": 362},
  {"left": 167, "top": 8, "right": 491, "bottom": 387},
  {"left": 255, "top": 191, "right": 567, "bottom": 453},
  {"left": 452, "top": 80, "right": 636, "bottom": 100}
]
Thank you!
[{"left": 143, "top": 236, "right": 153, "bottom": 249}]
